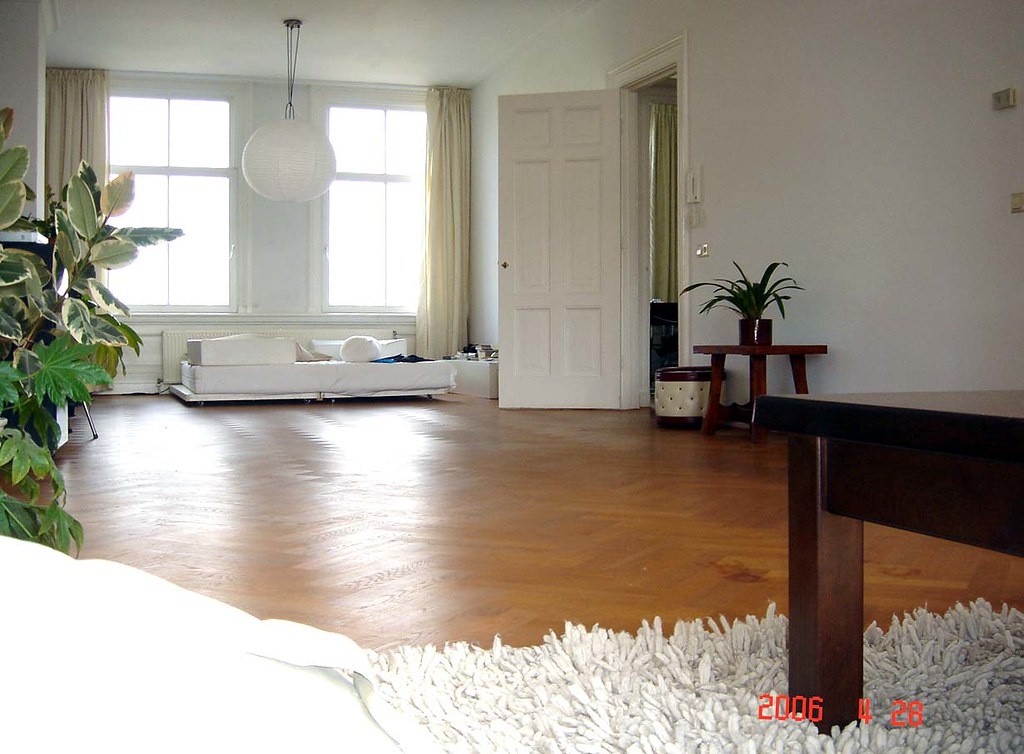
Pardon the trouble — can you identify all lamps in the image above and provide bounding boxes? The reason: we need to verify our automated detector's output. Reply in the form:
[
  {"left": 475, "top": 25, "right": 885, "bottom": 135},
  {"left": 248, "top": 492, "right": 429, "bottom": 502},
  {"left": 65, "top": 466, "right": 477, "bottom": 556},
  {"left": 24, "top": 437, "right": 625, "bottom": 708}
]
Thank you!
[{"left": 242, "top": 19, "right": 336, "bottom": 202}]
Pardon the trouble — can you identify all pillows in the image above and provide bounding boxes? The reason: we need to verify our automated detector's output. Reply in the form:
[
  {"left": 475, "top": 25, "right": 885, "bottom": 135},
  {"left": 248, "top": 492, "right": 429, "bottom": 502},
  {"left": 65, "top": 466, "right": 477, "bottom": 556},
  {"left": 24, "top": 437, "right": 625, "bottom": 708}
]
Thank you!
[{"left": 341, "top": 336, "right": 382, "bottom": 362}]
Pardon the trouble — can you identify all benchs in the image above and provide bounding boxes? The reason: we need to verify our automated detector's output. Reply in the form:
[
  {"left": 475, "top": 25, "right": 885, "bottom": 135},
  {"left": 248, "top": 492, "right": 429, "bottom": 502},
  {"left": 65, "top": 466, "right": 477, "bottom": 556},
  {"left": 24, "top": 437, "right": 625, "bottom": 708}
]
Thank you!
[{"left": 169, "top": 333, "right": 453, "bottom": 407}]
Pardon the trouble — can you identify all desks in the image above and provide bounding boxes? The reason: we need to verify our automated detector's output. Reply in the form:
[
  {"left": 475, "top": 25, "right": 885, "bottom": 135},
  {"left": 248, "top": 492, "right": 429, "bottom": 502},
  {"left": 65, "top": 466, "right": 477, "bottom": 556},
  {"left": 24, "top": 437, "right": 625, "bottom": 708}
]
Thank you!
[
  {"left": 693, "top": 345, "right": 827, "bottom": 443},
  {"left": 752, "top": 390, "right": 1023, "bottom": 735}
]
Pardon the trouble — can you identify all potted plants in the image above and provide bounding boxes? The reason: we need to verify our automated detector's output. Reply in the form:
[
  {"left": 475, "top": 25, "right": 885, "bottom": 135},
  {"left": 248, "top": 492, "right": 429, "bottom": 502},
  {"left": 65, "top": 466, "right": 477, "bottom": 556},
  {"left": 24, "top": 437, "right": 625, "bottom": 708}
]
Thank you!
[{"left": 680, "top": 261, "right": 806, "bottom": 345}]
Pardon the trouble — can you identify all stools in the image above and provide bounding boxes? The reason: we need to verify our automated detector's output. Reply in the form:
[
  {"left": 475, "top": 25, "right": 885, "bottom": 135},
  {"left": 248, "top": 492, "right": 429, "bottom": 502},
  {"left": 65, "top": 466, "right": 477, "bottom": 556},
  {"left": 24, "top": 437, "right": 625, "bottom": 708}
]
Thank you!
[{"left": 655, "top": 366, "right": 726, "bottom": 429}]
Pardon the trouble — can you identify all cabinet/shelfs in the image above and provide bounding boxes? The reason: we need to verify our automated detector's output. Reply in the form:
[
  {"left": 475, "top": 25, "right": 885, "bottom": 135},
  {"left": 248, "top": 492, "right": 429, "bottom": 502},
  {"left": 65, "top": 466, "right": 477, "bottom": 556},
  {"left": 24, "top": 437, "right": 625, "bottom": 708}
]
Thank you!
[{"left": 450, "top": 360, "right": 498, "bottom": 399}]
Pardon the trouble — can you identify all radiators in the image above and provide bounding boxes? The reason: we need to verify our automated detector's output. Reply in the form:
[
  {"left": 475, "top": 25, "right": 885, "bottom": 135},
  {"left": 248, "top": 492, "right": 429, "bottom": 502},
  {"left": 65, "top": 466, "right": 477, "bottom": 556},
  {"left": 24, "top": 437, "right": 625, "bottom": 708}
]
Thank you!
[{"left": 161, "top": 329, "right": 394, "bottom": 384}]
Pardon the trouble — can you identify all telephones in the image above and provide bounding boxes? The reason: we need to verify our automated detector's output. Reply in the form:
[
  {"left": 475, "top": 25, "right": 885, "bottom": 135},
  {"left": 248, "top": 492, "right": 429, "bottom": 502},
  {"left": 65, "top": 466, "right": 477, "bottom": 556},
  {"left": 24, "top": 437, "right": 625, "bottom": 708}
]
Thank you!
[{"left": 489, "top": 350, "right": 499, "bottom": 358}]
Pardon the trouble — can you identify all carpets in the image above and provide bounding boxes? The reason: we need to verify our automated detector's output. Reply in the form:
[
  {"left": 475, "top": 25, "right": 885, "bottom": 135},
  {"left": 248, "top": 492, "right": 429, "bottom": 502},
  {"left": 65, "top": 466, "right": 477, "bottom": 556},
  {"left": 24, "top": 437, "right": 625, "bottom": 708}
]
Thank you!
[{"left": 376, "top": 597, "right": 1024, "bottom": 754}]
